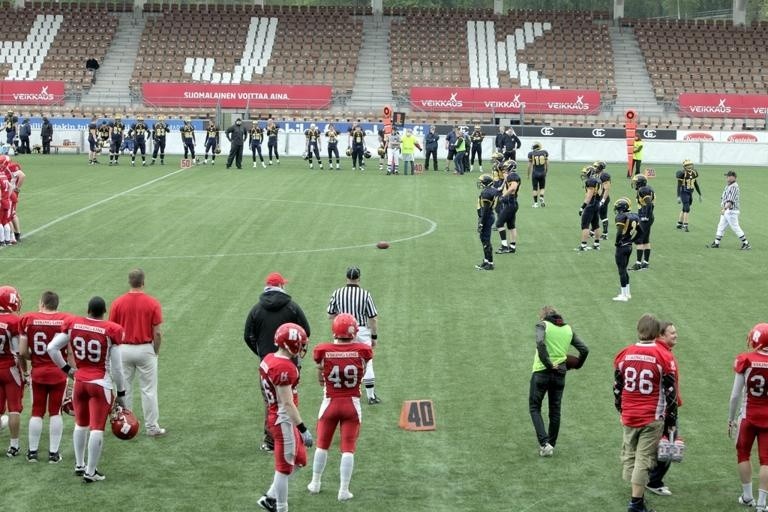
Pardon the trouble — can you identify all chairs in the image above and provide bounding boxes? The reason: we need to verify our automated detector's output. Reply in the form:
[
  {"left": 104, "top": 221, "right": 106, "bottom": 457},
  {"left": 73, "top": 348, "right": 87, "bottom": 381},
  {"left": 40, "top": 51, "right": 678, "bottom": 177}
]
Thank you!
[
  {"left": 618, "top": 15, "right": 768, "bottom": 108},
  {"left": 125, "top": 3, "right": 373, "bottom": 105},
  {"left": 0, "top": 104, "right": 216, "bottom": 121},
  {"left": 33, "top": 143, "right": 39, "bottom": 154},
  {"left": 407, "top": 112, "right": 491, "bottom": 124},
  {"left": 637, "top": 116, "right": 766, "bottom": 131},
  {"left": 382, "top": 6, "right": 618, "bottom": 108},
  {"left": 249, "top": 108, "right": 384, "bottom": 123},
  {"left": 523, "top": 113, "right": 626, "bottom": 128},
  {"left": 0, "top": 2, "right": 124, "bottom": 96}
]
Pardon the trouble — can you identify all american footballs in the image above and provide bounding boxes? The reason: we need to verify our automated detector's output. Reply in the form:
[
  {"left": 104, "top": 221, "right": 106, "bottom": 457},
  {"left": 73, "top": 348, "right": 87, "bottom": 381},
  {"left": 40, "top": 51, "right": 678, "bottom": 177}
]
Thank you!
[{"left": 375, "top": 240, "right": 391, "bottom": 250}]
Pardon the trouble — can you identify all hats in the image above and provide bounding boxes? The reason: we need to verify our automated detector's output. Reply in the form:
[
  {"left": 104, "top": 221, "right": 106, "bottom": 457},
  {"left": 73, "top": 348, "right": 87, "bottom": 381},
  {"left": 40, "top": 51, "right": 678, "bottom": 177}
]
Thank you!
[
  {"left": 346, "top": 264, "right": 360, "bottom": 280},
  {"left": 724, "top": 171, "right": 736, "bottom": 177},
  {"left": 266, "top": 273, "right": 288, "bottom": 286}
]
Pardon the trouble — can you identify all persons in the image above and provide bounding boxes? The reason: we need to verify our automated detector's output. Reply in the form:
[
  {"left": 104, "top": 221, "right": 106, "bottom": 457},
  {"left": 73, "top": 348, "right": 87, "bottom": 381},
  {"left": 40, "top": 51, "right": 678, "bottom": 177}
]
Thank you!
[
  {"left": 500, "top": 128, "right": 521, "bottom": 160},
  {"left": 634, "top": 134, "right": 643, "bottom": 173},
  {"left": 324, "top": 124, "right": 340, "bottom": 170},
  {"left": 711, "top": 171, "right": 752, "bottom": 250},
  {"left": 40, "top": 116, "right": 53, "bottom": 154},
  {"left": 97, "top": 120, "right": 108, "bottom": 146},
  {"left": 589, "top": 161, "right": 611, "bottom": 241},
  {"left": 612, "top": 316, "right": 678, "bottom": 512},
  {"left": 243, "top": 274, "right": 311, "bottom": 454},
  {"left": 645, "top": 322, "right": 682, "bottom": 497},
  {"left": 20, "top": 119, "right": 32, "bottom": 154},
  {"left": 612, "top": 199, "right": 645, "bottom": 303},
  {"left": 203, "top": 120, "right": 221, "bottom": 164},
  {"left": 249, "top": 120, "right": 267, "bottom": 169},
  {"left": 108, "top": 268, "right": 166, "bottom": 437},
  {"left": 225, "top": 118, "right": 248, "bottom": 170},
  {"left": 401, "top": 128, "right": 423, "bottom": 175},
  {"left": 151, "top": 115, "right": 170, "bottom": 166},
  {"left": 108, "top": 116, "right": 125, "bottom": 165},
  {"left": 0, "top": 285, "right": 29, "bottom": 457},
  {"left": 85, "top": 55, "right": 99, "bottom": 84},
  {"left": 255, "top": 322, "right": 313, "bottom": 512},
  {"left": 574, "top": 166, "right": 601, "bottom": 252},
  {"left": 470, "top": 125, "right": 485, "bottom": 172},
  {"left": 475, "top": 173, "right": 499, "bottom": 270},
  {"left": 327, "top": 267, "right": 384, "bottom": 403},
  {"left": 728, "top": 323, "right": 768, "bottom": 511},
  {"left": 451, "top": 131, "right": 466, "bottom": 175},
  {"left": 495, "top": 126, "right": 505, "bottom": 153},
  {"left": 128, "top": 117, "right": 151, "bottom": 166},
  {"left": 445, "top": 127, "right": 457, "bottom": 171},
  {"left": 0, "top": 155, "right": 23, "bottom": 247},
  {"left": 0, "top": 111, "right": 18, "bottom": 155},
  {"left": 424, "top": 126, "right": 439, "bottom": 172},
  {"left": 307, "top": 314, "right": 372, "bottom": 501},
  {"left": 628, "top": 173, "right": 656, "bottom": 271},
  {"left": 87, "top": 115, "right": 100, "bottom": 164},
  {"left": 180, "top": 117, "right": 198, "bottom": 167},
  {"left": 528, "top": 306, "right": 589, "bottom": 456},
  {"left": 349, "top": 127, "right": 367, "bottom": 170},
  {"left": 462, "top": 134, "right": 471, "bottom": 173},
  {"left": 377, "top": 129, "right": 389, "bottom": 170},
  {"left": 676, "top": 159, "right": 703, "bottom": 233},
  {"left": 265, "top": 119, "right": 281, "bottom": 165},
  {"left": 47, "top": 296, "right": 126, "bottom": 480},
  {"left": 383, "top": 127, "right": 401, "bottom": 174},
  {"left": 492, "top": 153, "right": 508, "bottom": 215},
  {"left": 495, "top": 160, "right": 522, "bottom": 254},
  {"left": 304, "top": 123, "right": 323, "bottom": 169},
  {"left": 528, "top": 141, "right": 549, "bottom": 210},
  {"left": 17, "top": 291, "right": 68, "bottom": 463},
  {"left": 347, "top": 121, "right": 362, "bottom": 133}
]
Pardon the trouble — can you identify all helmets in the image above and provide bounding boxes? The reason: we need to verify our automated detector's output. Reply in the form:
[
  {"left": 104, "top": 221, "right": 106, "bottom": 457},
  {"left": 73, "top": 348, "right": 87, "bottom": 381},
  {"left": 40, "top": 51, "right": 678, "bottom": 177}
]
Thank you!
[
  {"left": 302, "top": 152, "right": 309, "bottom": 160},
  {"left": 137, "top": 116, "right": 144, "bottom": 121},
  {"left": 364, "top": 151, "right": 371, "bottom": 158},
  {"left": 115, "top": 116, "right": 121, "bottom": 120},
  {"left": 491, "top": 152, "right": 504, "bottom": 163},
  {"left": 61, "top": 396, "right": 75, "bottom": 416},
  {"left": 580, "top": 166, "right": 596, "bottom": 180},
  {"left": 214, "top": 148, "right": 221, "bottom": 154},
  {"left": 593, "top": 160, "right": 606, "bottom": 173},
  {"left": 109, "top": 405, "right": 139, "bottom": 440},
  {"left": 477, "top": 174, "right": 493, "bottom": 190},
  {"left": 377, "top": 148, "right": 384, "bottom": 156},
  {"left": 746, "top": 323, "right": 768, "bottom": 351},
  {"left": 613, "top": 197, "right": 632, "bottom": 214},
  {"left": 332, "top": 313, "right": 359, "bottom": 339},
  {"left": 274, "top": 323, "right": 309, "bottom": 359},
  {"left": 0, "top": 285, "right": 23, "bottom": 313},
  {"left": 252, "top": 120, "right": 258, "bottom": 124},
  {"left": 682, "top": 160, "right": 694, "bottom": 171},
  {"left": 502, "top": 160, "right": 517, "bottom": 174},
  {"left": 8, "top": 108, "right": 14, "bottom": 113},
  {"left": 531, "top": 141, "right": 542, "bottom": 150},
  {"left": 346, "top": 148, "right": 352, "bottom": 157},
  {"left": 473, "top": 125, "right": 481, "bottom": 131},
  {"left": 184, "top": 117, "right": 191, "bottom": 122},
  {"left": 630, "top": 174, "right": 648, "bottom": 190},
  {"left": 159, "top": 116, "right": 164, "bottom": 121}
]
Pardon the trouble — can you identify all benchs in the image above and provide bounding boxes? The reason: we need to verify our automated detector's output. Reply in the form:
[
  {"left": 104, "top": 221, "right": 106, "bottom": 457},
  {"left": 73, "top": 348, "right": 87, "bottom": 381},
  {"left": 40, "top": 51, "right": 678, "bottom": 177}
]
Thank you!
[{"left": 57, "top": 144, "right": 81, "bottom": 155}]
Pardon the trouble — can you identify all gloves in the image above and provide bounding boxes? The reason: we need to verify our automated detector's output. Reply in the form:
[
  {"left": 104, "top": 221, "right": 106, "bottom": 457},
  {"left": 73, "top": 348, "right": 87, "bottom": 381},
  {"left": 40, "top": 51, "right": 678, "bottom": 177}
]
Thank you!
[
  {"left": 599, "top": 201, "right": 604, "bottom": 208},
  {"left": 61, "top": 364, "right": 76, "bottom": 380},
  {"left": 114, "top": 390, "right": 126, "bottom": 409},
  {"left": 300, "top": 429, "right": 313, "bottom": 448},
  {"left": 728, "top": 420, "right": 737, "bottom": 438},
  {"left": 578, "top": 207, "right": 584, "bottom": 216},
  {"left": 23, "top": 371, "right": 30, "bottom": 387},
  {"left": 499, "top": 196, "right": 504, "bottom": 202},
  {"left": 477, "top": 224, "right": 484, "bottom": 234},
  {"left": 677, "top": 197, "right": 681, "bottom": 204},
  {"left": 699, "top": 196, "right": 703, "bottom": 202}
]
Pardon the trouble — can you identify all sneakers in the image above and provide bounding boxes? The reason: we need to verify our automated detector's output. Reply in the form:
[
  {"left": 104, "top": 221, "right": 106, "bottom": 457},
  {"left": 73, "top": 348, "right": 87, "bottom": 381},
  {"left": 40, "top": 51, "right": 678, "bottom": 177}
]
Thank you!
[
  {"left": 479, "top": 166, "right": 483, "bottom": 173},
  {"left": 259, "top": 443, "right": 273, "bottom": 454},
  {"left": 131, "top": 161, "right": 135, "bottom": 167},
  {"left": 15, "top": 232, "right": 21, "bottom": 240},
  {"left": 203, "top": 159, "right": 207, "bottom": 164},
  {"left": 329, "top": 163, "right": 333, "bottom": 170},
  {"left": 25, "top": 453, "right": 38, "bottom": 463},
  {"left": 6, "top": 446, "right": 21, "bottom": 458},
  {"left": 192, "top": 159, "right": 195, "bottom": 164},
  {"left": 261, "top": 162, "right": 267, "bottom": 169},
  {"left": 645, "top": 479, "right": 672, "bottom": 496},
  {"left": 627, "top": 499, "right": 656, "bottom": 512},
  {"left": 109, "top": 160, "right": 113, "bottom": 166},
  {"left": 540, "top": 450, "right": 553, "bottom": 456},
  {"left": 495, "top": 245, "right": 509, "bottom": 254},
  {"left": 641, "top": 262, "right": 649, "bottom": 270},
  {"left": 114, "top": 160, "right": 118, "bottom": 165},
  {"left": 352, "top": 166, "right": 356, "bottom": 170},
  {"left": 599, "top": 234, "right": 608, "bottom": 241},
  {"left": 276, "top": 160, "right": 280, "bottom": 164},
  {"left": 307, "top": 481, "right": 321, "bottom": 494},
  {"left": 617, "top": 294, "right": 631, "bottom": 299},
  {"left": 470, "top": 165, "right": 474, "bottom": 172},
  {"left": 587, "top": 243, "right": 600, "bottom": 251},
  {"left": 387, "top": 170, "right": 391, "bottom": 175},
  {"left": 74, "top": 464, "right": 97, "bottom": 475},
  {"left": 359, "top": 167, "right": 364, "bottom": 171},
  {"left": 211, "top": 160, "right": 215, "bottom": 164},
  {"left": 395, "top": 170, "right": 399, "bottom": 175},
  {"left": 741, "top": 242, "right": 752, "bottom": 249},
  {"left": 82, "top": 470, "right": 105, "bottom": 483},
  {"left": 88, "top": 160, "right": 92, "bottom": 165},
  {"left": 539, "top": 197, "right": 545, "bottom": 207},
  {"left": 147, "top": 427, "right": 166, "bottom": 436},
  {"left": 588, "top": 231, "right": 595, "bottom": 238},
  {"left": 612, "top": 296, "right": 628, "bottom": 302},
  {"left": 92, "top": 161, "right": 100, "bottom": 165},
  {"left": 475, "top": 259, "right": 487, "bottom": 268},
  {"left": 475, "top": 264, "right": 495, "bottom": 271},
  {"left": 309, "top": 163, "right": 313, "bottom": 169},
  {"left": 0, "top": 241, "right": 6, "bottom": 248},
  {"left": 160, "top": 159, "right": 163, "bottom": 165},
  {"left": 683, "top": 224, "right": 688, "bottom": 232},
  {"left": 256, "top": 494, "right": 277, "bottom": 512},
  {"left": 253, "top": 162, "right": 257, "bottom": 168},
  {"left": 336, "top": 163, "right": 340, "bottom": 169},
  {"left": 738, "top": 495, "right": 756, "bottom": 507},
  {"left": 269, "top": 161, "right": 272, "bottom": 165},
  {"left": 379, "top": 165, "right": 383, "bottom": 170},
  {"left": 539, "top": 444, "right": 553, "bottom": 456},
  {"left": 574, "top": 244, "right": 587, "bottom": 252},
  {"left": 676, "top": 222, "right": 683, "bottom": 230},
  {"left": 628, "top": 264, "right": 642, "bottom": 272},
  {"left": 508, "top": 244, "right": 515, "bottom": 253},
  {"left": 10, "top": 239, "right": 17, "bottom": 244},
  {"left": 5, "top": 240, "right": 12, "bottom": 246},
  {"left": 532, "top": 203, "right": 538, "bottom": 208},
  {"left": 710, "top": 241, "right": 719, "bottom": 248},
  {"left": 142, "top": 161, "right": 146, "bottom": 166},
  {"left": 319, "top": 164, "right": 323, "bottom": 169},
  {"left": 755, "top": 505, "right": 768, "bottom": 512},
  {"left": 337, "top": 490, "right": 353, "bottom": 501},
  {"left": 368, "top": 393, "right": 380, "bottom": 404},
  {"left": 48, "top": 451, "right": 62, "bottom": 464},
  {"left": 150, "top": 160, "right": 155, "bottom": 165}
]
